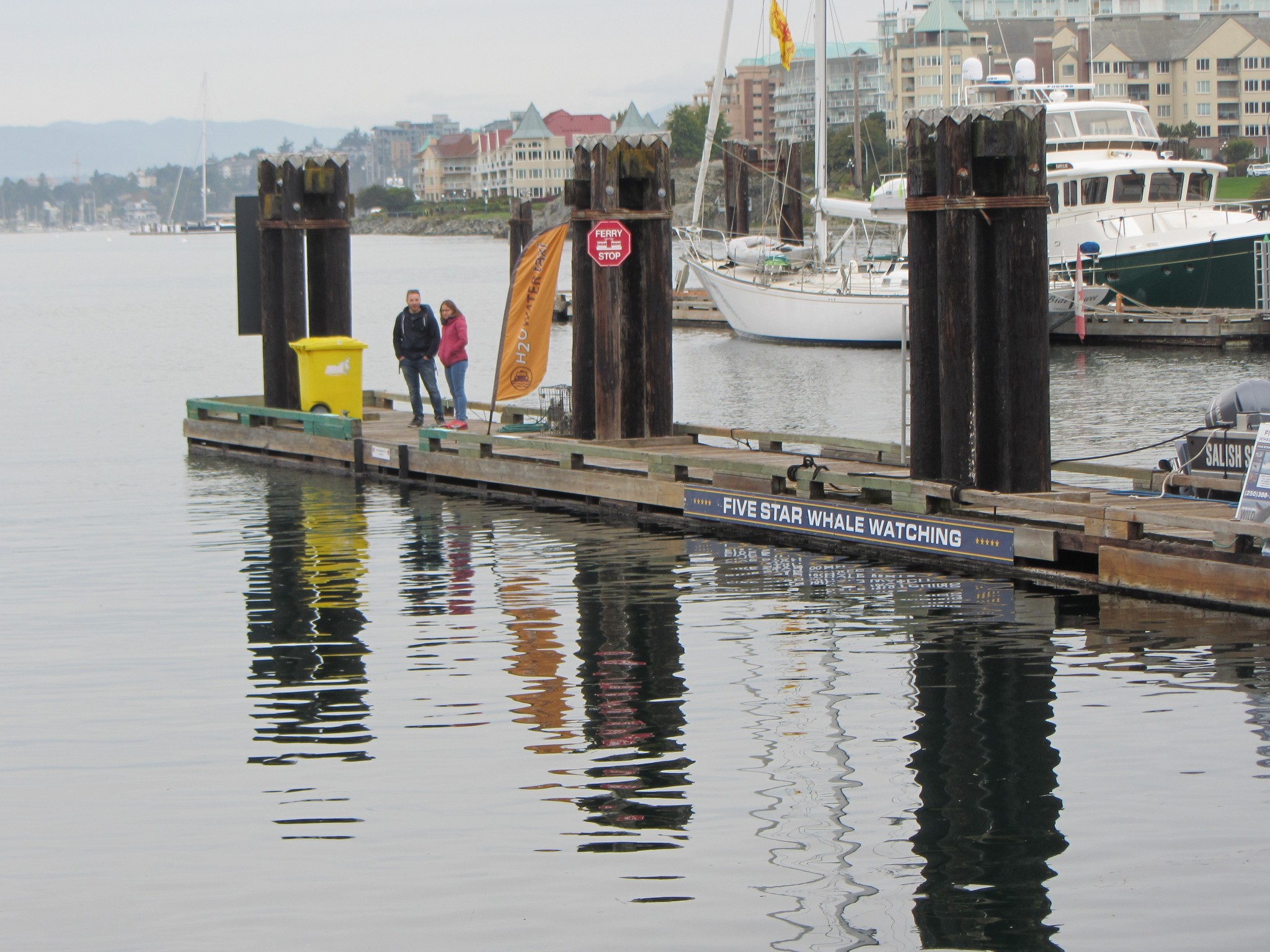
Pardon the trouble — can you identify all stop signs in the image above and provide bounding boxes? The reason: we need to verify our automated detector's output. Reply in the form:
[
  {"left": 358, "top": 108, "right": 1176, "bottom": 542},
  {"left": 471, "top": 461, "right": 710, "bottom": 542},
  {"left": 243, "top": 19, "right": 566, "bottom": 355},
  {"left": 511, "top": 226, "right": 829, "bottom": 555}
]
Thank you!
[{"left": 588, "top": 220, "right": 631, "bottom": 267}]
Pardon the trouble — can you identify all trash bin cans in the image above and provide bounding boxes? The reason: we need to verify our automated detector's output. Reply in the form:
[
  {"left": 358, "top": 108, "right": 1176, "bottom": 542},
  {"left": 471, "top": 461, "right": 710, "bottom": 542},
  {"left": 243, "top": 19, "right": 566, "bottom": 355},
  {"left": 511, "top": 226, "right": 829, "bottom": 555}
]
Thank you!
[{"left": 289, "top": 335, "right": 368, "bottom": 422}]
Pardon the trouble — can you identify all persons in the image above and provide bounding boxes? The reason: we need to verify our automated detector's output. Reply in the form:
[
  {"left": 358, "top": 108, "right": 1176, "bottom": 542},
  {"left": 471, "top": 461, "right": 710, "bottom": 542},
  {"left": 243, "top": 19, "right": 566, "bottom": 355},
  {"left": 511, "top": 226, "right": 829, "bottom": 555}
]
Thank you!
[
  {"left": 438, "top": 299, "right": 470, "bottom": 430},
  {"left": 392, "top": 289, "right": 448, "bottom": 428}
]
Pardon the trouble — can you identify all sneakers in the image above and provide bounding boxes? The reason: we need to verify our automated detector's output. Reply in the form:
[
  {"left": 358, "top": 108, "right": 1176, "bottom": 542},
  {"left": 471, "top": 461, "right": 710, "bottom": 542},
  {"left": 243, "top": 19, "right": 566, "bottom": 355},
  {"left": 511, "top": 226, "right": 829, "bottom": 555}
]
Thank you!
[
  {"left": 444, "top": 419, "right": 468, "bottom": 430},
  {"left": 407, "top": 415, "right": 424, "bottom": 427},
  {"left": 434, "top": 414, "right": 446, "bottom": 427}
]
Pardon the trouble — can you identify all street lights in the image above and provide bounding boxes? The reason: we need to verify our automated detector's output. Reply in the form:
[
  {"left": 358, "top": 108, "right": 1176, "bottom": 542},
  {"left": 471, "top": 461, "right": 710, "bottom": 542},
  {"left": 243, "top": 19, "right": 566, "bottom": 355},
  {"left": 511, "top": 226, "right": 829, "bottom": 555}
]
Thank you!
[{"left": 847, "top": 134, "right": 868, "bottom": 178}]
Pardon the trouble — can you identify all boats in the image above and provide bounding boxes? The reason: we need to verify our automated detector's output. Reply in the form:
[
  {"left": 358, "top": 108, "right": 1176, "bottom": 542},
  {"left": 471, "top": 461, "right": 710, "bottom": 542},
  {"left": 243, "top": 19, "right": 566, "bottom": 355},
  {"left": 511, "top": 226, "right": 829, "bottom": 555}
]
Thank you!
[{"left": 866, "top": 56, "right": 1269, "bottom": 314}]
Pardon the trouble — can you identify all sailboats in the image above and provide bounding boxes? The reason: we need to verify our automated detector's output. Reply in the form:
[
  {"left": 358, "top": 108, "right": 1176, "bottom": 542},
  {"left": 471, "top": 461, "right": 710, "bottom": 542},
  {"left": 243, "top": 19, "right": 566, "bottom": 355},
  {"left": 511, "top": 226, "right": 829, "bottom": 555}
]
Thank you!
[{"left": 671, "top": 0, "right": 1113, "bottom": 345}]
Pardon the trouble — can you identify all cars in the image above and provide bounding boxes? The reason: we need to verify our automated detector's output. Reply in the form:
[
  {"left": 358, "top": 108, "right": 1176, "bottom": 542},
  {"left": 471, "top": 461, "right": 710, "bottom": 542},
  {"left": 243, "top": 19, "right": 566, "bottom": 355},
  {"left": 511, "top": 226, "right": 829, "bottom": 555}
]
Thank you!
[{"left": 1246, "top": 163, "right": 1270, "bottom": 177}]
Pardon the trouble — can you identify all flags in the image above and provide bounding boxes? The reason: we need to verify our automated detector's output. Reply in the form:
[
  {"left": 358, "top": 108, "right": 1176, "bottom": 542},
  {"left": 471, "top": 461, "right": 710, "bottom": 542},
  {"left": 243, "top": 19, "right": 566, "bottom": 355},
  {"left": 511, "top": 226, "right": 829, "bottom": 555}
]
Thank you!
[
  {"left": 1073, "top": 246, "right": 1086, "bottom": 341},
  {"left": 769, "top": 0, "right": 796, "bottom": 72}
]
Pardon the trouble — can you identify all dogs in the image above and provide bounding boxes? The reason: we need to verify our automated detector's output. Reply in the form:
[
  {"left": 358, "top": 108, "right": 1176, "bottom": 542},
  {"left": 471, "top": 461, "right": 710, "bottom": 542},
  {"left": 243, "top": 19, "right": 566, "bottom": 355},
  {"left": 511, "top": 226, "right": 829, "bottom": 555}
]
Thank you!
[{"left": 547, "top": 397, "right": 565, "bottom": 432}]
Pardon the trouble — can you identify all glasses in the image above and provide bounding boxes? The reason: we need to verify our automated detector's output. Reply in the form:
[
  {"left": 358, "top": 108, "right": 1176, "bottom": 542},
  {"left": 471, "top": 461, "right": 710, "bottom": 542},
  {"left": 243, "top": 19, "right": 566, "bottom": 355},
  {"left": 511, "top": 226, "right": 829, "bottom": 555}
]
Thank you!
[
  {"left": 407, "top": 290, "right": 419, "bottom": 293},
  {"left": 441, "top": 309, "right": 450, "bottom": 312}
]
